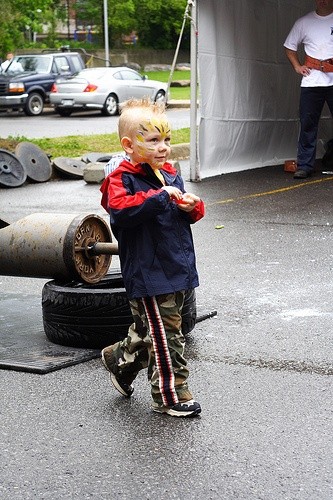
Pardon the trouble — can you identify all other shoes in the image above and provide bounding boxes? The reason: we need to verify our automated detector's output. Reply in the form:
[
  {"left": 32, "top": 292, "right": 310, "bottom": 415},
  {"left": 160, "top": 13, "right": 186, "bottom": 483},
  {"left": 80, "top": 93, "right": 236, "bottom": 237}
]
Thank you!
[
  {"left": 101, "top": 345, "right": 134, "bottom": 397},
  {"left": 294, "top": 171, "right": 317, "bottom": 180},
  {"left": 152, "top": 400, "right": 201, "bottom": 417}
]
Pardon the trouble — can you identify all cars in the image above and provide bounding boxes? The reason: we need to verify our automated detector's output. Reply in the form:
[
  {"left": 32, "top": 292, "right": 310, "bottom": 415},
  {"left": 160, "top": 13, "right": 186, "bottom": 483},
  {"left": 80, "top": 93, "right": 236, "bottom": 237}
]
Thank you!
[{"left": 49, "top": 66, "right": 169, "bottom": 117}]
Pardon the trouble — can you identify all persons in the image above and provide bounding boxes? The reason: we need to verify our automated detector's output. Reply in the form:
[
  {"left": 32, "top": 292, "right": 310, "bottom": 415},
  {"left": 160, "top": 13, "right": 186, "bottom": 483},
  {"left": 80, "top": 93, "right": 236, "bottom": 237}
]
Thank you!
[
  {"left": 100, "top": 98, "right": 205, "bottom": 416},
  {"left": 284, "top": 0, "right": 333, "bottom": 178},
  {"left": 0, "top": 52, "right": 24, "bottom": 75}
]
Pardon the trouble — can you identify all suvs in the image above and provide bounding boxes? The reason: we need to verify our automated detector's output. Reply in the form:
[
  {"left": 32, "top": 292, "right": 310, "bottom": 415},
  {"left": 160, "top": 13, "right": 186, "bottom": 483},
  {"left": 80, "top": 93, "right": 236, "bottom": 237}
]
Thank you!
[{"left": 0, "top": 52, "right": 86, "bottom": 116}]
